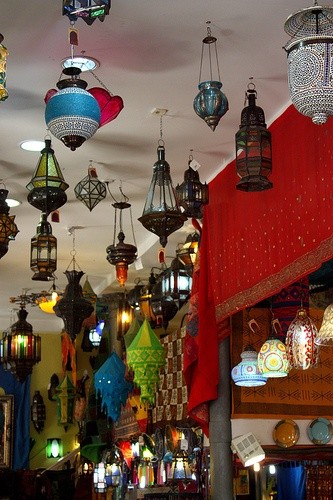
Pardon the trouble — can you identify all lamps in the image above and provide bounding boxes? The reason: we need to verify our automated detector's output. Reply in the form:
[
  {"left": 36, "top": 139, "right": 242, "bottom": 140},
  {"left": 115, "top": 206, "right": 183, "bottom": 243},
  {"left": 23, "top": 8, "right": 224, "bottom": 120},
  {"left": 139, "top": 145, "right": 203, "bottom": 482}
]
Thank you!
[{"left": 0, "top": 0, "right": 333, "bottom": 493}]
